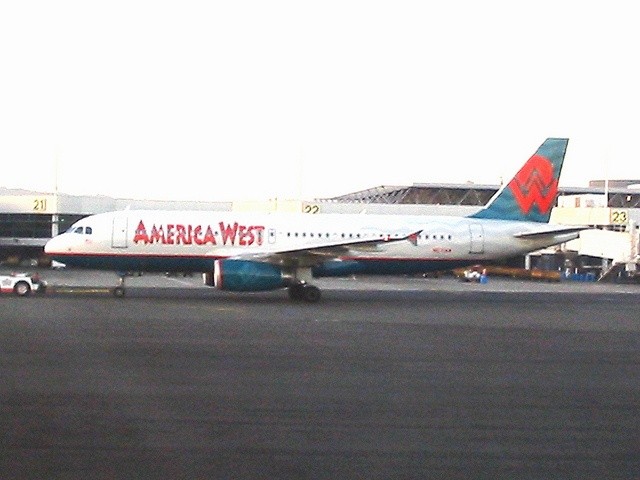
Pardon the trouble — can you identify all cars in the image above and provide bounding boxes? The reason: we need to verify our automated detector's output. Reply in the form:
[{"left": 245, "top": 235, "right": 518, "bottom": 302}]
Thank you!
[{"left": 0, "top": 271, "right": 48, "bottom": 297}]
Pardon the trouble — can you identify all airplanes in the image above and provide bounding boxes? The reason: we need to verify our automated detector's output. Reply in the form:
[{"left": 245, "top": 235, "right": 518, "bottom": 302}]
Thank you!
[{"left": 44, "top": 137, "right": 591, "bottom": 302}]
